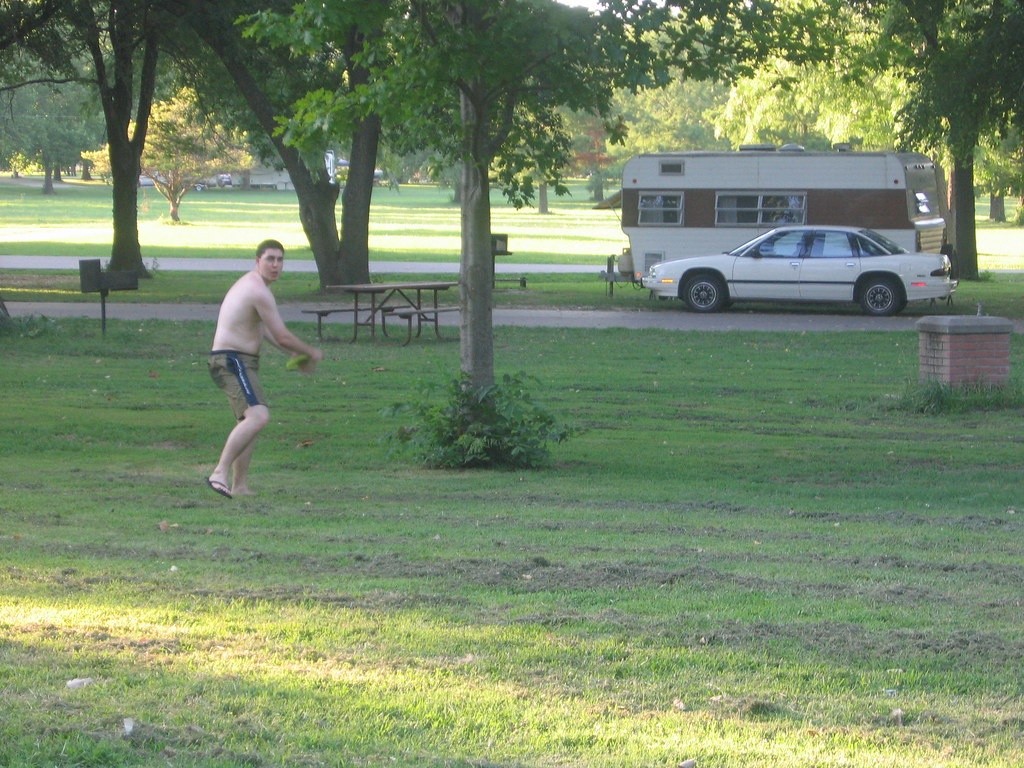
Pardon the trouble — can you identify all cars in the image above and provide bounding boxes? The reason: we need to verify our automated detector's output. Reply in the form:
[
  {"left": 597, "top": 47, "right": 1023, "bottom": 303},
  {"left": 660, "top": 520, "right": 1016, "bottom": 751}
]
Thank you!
[
  {"left": 137, "top": 173, "right": 232, "bottom": 191},
  {"left": 639, "top": 223, "right": 959, "bottom": 318}
]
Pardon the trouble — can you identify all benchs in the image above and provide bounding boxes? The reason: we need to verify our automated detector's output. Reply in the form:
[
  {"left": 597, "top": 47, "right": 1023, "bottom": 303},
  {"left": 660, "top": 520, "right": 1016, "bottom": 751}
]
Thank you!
[
  {"left": 301, "top": 305, "right": 410, "bottom": 343},
  {"left": 383, "top": 307, "right": 459, "bottom": 345},
  {"left": 492, "top": 277, "right": 528, "bottom": 288}
]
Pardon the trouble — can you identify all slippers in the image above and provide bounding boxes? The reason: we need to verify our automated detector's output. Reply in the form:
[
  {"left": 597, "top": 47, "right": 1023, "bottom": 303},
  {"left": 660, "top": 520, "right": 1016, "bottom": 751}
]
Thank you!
[{"left": 207, "top": 478, "right": 232, "bottom": 498}]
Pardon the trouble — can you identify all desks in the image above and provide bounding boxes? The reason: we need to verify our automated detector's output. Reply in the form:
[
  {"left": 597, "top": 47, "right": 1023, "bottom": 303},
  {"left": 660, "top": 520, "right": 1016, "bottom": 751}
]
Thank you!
[{"left": 327, "top": 282, "right": 459, "bottom": 344}]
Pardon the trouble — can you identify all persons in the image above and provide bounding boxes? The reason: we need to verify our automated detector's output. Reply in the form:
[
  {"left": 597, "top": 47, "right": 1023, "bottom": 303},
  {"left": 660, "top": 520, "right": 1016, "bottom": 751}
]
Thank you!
[{"left": 204, "top": 240, "right": 322, "bottom": 497}]
